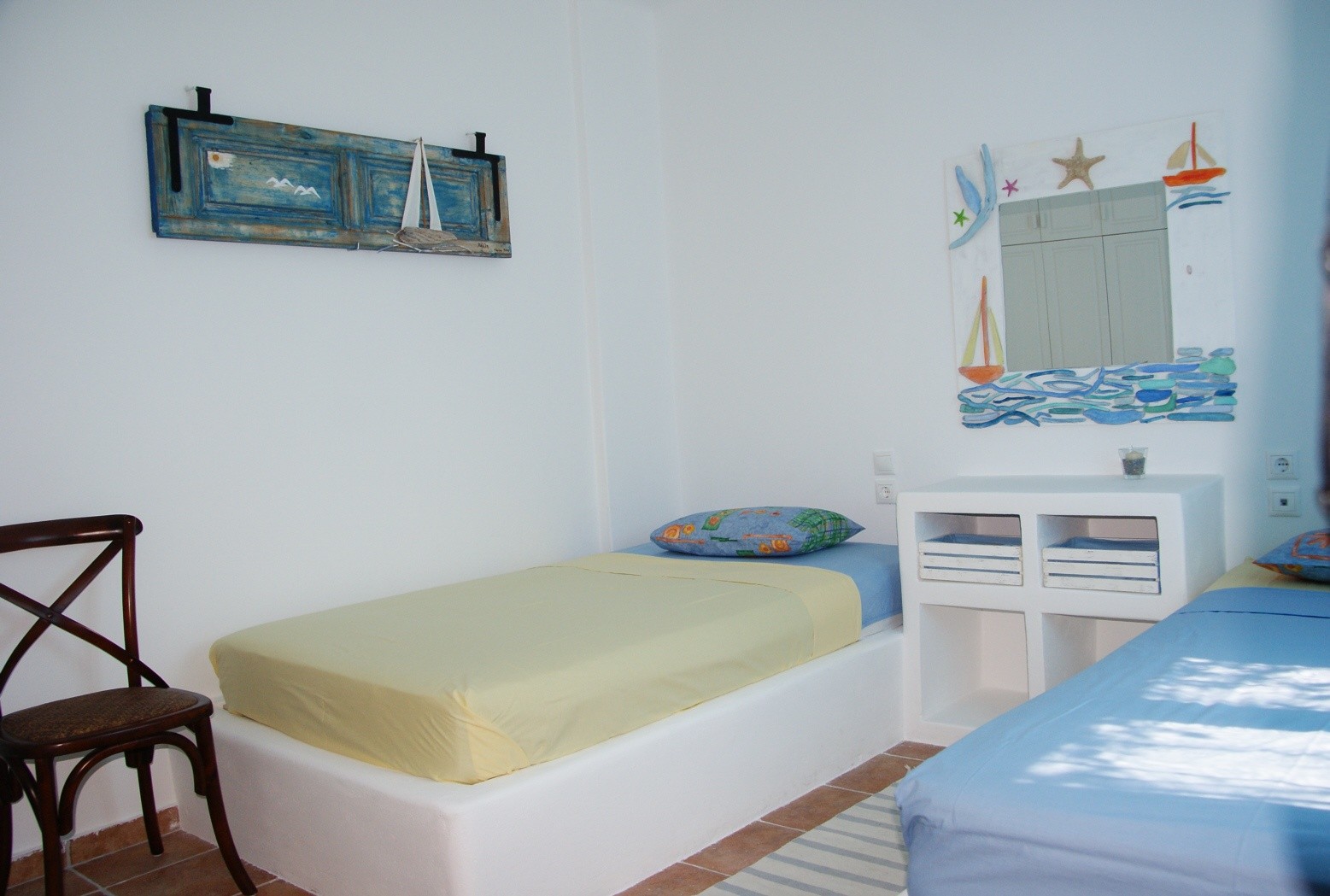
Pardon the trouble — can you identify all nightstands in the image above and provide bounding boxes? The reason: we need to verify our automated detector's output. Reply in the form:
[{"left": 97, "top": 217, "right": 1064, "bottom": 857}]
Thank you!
[{"left": 896, "top": 475, "right": 1223, "bottom": 751}]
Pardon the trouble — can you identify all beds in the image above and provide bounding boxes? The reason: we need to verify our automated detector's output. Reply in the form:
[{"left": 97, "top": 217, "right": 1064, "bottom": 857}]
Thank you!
[
  {"left": 179, "top": 537, "right": 906, "bottom": 896},
  {"left": 895, "top": 554, "right": 1330, "bottom": 896}
]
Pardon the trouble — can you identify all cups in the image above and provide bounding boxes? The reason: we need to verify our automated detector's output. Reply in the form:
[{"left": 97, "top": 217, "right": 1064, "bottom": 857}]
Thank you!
[{"left": 1118, "top": 448, "right": 1147, "bottom": 479}]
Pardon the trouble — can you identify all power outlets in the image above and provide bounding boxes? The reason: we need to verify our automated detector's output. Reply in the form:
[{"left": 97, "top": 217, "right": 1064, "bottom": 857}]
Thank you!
[
  {"left": 1265, "top": 450, "right": 1299, "bottom": 480},
  {"left": 874, "top": 479, "right": 899, "bottom": 505}
]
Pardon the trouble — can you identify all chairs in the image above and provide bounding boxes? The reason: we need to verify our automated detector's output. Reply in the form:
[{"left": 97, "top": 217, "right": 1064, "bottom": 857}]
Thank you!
[{"left": 0, "top": 514, "right": 259, "bottom": 896}]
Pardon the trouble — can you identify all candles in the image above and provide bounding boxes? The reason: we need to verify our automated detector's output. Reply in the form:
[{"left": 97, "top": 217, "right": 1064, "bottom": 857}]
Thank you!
[{"left": 1117, "top": 444, "right": 1149, "bottom": 479}]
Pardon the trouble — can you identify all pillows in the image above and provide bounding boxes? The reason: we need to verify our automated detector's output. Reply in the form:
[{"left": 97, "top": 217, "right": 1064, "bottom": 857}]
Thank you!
[
  {"left": 648, "top": 507, "right": 865, "bottom": 560},
  {"left": 1252, "top": 526, "right": 1330, "bottom": 586}
]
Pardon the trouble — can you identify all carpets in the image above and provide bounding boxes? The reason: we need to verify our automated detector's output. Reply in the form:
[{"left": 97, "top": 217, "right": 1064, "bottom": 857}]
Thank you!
[{"left": 696, "top": 777, "right": 910, "bottom": 896}]
